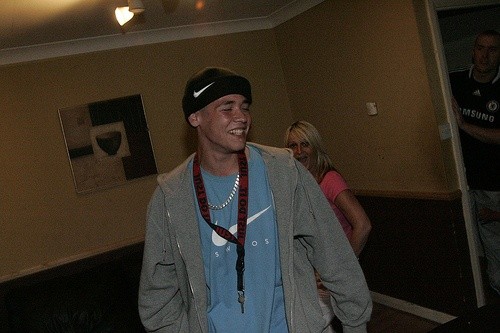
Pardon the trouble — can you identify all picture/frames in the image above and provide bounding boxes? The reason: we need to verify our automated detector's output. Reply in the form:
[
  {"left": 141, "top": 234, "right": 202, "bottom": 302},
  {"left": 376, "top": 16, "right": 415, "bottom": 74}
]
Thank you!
[{"left": 59, "top": 95, "right": 159, "bottom": 194}]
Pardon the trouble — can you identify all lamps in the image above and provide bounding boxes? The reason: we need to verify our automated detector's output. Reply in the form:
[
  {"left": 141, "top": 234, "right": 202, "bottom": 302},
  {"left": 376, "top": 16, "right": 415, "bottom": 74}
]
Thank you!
[{"left": 114, "top": 0, "right": 144, "bottom": 26}]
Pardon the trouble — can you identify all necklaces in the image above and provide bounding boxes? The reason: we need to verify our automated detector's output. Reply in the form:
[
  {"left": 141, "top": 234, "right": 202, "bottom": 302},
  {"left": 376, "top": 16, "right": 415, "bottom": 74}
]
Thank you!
[{"left": 208, "top": 171, "right": 239, "bottom": 210}]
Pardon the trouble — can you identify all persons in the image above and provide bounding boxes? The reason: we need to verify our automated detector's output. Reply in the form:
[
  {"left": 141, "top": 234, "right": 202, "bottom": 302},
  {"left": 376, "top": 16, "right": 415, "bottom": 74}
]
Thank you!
[
  {"left": 138, "top": 66, "right": 373, "bottom": 333},
  {"left": 283, "top": 121, "right": 372, "bottom": 333},
  {"left": 450, "top": 32, "right": 500, "bottom": 314}
]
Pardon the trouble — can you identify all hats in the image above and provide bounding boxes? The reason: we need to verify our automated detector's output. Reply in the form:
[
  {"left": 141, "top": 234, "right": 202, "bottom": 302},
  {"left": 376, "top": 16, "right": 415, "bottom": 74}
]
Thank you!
[{"left": 182, "top": 65, "right": 252, "bottom": 128}]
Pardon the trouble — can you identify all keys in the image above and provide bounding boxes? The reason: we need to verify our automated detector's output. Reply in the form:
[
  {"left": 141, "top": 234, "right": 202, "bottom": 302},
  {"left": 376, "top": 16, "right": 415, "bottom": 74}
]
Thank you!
[{"left": 238, "top": 293, "right": 246, "bottom": 313}]
